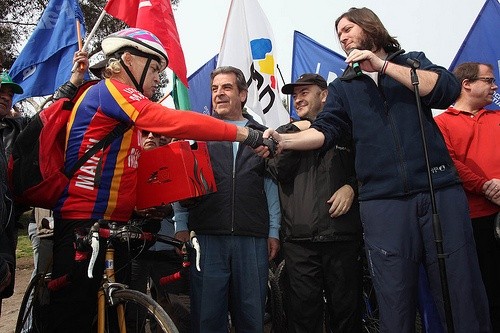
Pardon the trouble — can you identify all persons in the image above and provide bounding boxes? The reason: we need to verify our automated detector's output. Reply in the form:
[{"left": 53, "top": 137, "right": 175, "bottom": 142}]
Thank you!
[
  {"left": 433, "top": 61, "right": 500, "bottom": 333},
  {"left": 248, "top": 7, "right": 491, "bottom": 333},
  {"left": 268, "top": 73, "right": 363, "bottom": 333},
  {"left": 185, "top": 66, "right": 278, "bottom": 333},
  {"left": 0, "top": 49, "right": 185, "bottom": 333},
  {"left": 59, "top": 27, "right": 279, "bottom": 333}
]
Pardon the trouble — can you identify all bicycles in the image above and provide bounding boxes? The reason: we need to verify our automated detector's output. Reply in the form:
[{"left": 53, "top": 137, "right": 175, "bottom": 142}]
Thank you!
[{"left": 14, "top": 215, "right": 197, "bottom": 333}]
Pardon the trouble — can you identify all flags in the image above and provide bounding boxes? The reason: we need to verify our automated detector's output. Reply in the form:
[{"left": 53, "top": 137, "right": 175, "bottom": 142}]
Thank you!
[
  {"left": 8, "top": 0, "right": 91, "bottom": 108},
  {"left": 104, "top": 0, "right": 190, "bottom": 89},
  {"left": 216, "top": 0, "right": 289, "bottom": 131}
]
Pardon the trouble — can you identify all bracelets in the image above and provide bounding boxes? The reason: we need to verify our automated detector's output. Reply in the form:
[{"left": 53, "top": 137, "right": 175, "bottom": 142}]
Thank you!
[{"left": 380, "top": 60, "right": 388, "bottom": 75}]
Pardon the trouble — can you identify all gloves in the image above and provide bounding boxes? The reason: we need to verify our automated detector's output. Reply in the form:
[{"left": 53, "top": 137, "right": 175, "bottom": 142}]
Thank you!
[
  {"left": 242, "top": 125, "right": 280, "bottom": 160},
  {"left": 145, "top": 203, "right": 174, "bottom": 221}
]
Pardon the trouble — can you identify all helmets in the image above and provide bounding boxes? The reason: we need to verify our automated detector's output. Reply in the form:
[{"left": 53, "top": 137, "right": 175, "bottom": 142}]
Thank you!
[{"left": 101, "top": 27, "right": 169, "bottom": 71}]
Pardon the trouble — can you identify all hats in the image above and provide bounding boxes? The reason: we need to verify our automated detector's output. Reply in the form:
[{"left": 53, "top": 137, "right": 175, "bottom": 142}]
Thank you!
[
  {"left": 0, "top": 71, "right": 24, "bottom": 94},
  {"left": 282, "top": 74, "right": 327, "bottom": 95}
]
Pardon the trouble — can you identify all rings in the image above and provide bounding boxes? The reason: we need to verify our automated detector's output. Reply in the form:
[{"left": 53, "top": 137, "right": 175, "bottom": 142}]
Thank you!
[{"left": 494, "top": 187, "right": 498, "bottom": 191}]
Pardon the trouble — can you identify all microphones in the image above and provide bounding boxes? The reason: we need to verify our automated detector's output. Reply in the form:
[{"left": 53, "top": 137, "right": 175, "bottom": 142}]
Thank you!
[{"left": 349, "top": 49, "right": 362, "bottom": 76}]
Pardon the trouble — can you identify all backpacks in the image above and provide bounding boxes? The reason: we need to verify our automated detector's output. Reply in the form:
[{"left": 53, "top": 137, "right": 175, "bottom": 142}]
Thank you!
[{"left": 8, "top": 79, "right": 132, "bottom": 211}]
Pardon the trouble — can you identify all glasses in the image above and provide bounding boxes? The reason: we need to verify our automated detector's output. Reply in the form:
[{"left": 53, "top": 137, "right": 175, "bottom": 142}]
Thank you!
[{"left": 469, "top": 77, "right": 495, "bottom": 85}]
